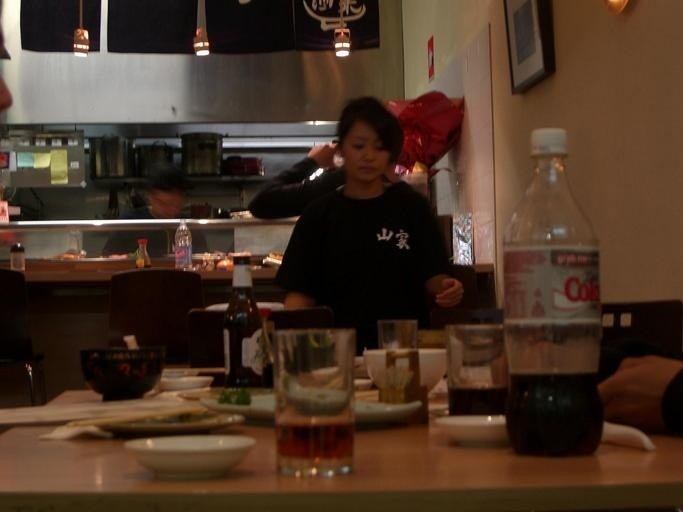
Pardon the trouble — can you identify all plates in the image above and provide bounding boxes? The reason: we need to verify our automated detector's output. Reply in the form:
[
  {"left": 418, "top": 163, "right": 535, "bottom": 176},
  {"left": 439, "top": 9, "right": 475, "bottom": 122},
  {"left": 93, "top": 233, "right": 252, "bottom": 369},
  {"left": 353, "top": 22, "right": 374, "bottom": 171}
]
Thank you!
[
  {"left": 161, "top": 374, "right": 210, "bottom": 391},
  {"left": 203, "top": 393, "right": 417, "bottom": 426},
  {"left": 122, "top": 435, "right": 259, "bottom": 484},
  {"left": 99, "top": 415, "right": 246, "bottom": 436},
  {"left": 432, "top": 417, "right": 510, "bottom": 446}
]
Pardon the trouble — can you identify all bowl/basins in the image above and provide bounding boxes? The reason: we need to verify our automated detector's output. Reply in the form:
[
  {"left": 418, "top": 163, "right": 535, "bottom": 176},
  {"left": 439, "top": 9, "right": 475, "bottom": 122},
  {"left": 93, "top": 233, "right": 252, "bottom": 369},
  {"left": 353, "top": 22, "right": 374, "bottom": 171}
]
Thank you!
[
  {"left": 75, "top": 346, "right": 172, "bottom": 399},
  {"left": 362, "top": 346, "right": 447, "bottom": 393}
]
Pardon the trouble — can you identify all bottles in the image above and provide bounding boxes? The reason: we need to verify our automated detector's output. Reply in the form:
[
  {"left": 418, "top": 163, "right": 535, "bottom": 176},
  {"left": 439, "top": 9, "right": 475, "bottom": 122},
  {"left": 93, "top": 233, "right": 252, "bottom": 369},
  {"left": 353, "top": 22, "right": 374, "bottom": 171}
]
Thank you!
[
  {"left": 222, "top": 257, "right": 264, "bottom": 382},
  {"left": 170, "top": 217, "right": 193, "bottom": 271},
  {"left": 500, "top": 125, "right": 608, "bottom": 462},
  {"left": 136, "top": 238, "right": 151, "bottom": 269},
  {"left": 9, "top": 242, "right": 26, "bottom": 272}
]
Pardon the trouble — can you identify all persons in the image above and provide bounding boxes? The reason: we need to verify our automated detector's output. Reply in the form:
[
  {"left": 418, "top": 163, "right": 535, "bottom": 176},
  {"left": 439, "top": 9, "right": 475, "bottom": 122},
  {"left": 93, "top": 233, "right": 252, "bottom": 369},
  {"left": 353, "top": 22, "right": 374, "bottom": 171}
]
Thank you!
[
  {"left": 248, "top": 136, "right": 452, "bottom": 277},
  {"left": 248, "top": 97, "right": 464, "bottom": 310},
  {"left": 101, "top": 162, "right": 208, "bottom": 255},
  {"left": 598, "top": 348, "right": 683, "bottom": 441}
]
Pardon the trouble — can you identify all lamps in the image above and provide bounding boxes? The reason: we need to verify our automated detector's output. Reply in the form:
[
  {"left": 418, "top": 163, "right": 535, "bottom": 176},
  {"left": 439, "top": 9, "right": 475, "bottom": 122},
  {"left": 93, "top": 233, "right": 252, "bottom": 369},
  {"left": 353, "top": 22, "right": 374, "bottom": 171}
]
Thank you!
[{"left": 73, "top": 0, "right": 351, "bottom": 62}]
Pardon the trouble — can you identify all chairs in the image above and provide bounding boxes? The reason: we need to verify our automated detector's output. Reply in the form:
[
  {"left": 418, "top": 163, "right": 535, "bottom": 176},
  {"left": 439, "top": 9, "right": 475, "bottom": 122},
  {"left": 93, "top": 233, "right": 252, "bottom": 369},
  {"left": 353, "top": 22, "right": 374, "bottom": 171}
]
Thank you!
[
  {"left": 105, "top": 269, "right": 199, "bottom": 339},
  {"left": 187, "top": 306, "right": 336, "bottom": 386},
  {"left": 0, "top": 268, "right": 45, "bottom": 404}
]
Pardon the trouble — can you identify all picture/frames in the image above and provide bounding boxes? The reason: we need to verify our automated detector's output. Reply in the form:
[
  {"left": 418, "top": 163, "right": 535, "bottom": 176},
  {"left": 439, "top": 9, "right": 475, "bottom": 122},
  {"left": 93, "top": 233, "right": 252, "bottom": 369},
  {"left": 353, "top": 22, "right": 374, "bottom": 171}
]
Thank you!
[{"left": 502, "top": 1, "right": 556, "bottom": 96}]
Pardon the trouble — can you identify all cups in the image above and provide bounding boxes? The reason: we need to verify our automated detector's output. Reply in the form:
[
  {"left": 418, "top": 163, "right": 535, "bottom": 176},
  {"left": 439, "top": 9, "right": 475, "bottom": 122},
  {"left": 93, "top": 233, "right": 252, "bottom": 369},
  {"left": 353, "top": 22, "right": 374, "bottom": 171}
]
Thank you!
[
  {"left": 271, "top": 326, "right": 361, "bottom": 480},
  {"left": 376, "top": 317, "right": 420, "bottom": 400},
  {"left": 441, "top": 319, "right": 507, "bottom": 414}
]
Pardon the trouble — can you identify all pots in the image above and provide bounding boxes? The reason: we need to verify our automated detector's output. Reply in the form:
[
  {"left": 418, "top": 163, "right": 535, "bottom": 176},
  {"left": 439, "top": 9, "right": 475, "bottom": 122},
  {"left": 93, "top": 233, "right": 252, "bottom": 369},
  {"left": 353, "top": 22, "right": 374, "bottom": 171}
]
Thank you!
[{"left": 86, "top": 131, "right": 231, "bottom": 177}]
